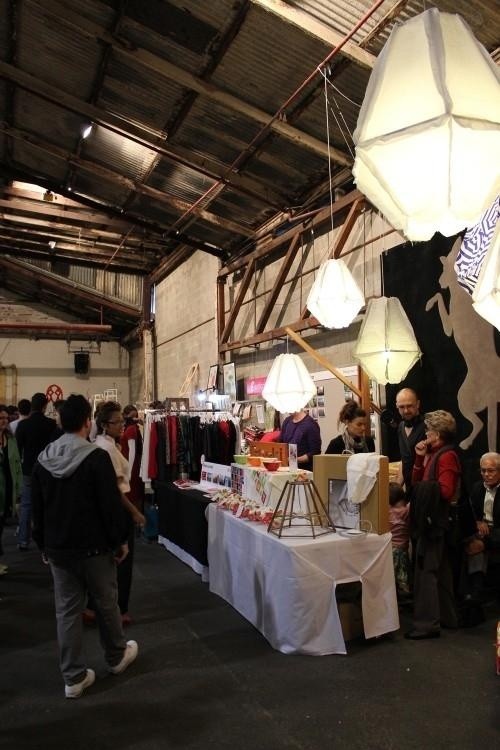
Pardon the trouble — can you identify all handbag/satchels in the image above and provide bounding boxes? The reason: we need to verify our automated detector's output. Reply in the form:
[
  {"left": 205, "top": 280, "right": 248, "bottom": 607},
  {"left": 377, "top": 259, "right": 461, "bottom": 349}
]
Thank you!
[{"left": 437, "top": 489, "right": 479, "bottom": 547}]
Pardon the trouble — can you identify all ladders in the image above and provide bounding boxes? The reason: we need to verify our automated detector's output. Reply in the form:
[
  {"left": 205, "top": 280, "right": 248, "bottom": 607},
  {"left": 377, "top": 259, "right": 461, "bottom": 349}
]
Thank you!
[{"left": 94, "top": 393, "right": 104, "bottom": 412}]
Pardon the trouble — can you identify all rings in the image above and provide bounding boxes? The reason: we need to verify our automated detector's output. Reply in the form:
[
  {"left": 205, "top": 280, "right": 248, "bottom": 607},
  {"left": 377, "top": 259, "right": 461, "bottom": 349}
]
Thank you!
[{"left": 423, "top": 445, "right": 425, "bottom": 447}]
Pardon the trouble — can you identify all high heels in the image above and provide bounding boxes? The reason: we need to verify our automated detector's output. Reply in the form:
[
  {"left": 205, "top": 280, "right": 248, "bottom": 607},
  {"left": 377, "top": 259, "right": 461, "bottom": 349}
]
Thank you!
[{"left": 404, "top": 620, "right": 441, "bottom": 640}]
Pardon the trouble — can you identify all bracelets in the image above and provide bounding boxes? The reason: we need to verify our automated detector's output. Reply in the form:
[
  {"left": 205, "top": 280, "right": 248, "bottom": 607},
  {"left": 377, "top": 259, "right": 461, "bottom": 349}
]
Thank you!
[{"left": 125, "top": 501, "right": 134, "bottom": 511}]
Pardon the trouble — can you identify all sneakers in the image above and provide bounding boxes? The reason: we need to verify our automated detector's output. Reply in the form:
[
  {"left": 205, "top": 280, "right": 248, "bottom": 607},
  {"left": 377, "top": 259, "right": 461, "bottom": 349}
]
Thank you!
[
  {"left": 110, "top": 640, "right": 139, "bottom": 674},
  {"left": 65, "top": 669, "right": 95, "bottom": 698},
  {"left": 82, "top": 607, "right": 133, "bottom": 627}
]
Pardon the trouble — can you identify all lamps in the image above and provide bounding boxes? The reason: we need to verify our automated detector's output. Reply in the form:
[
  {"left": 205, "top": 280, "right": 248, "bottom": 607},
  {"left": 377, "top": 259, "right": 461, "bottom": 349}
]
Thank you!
[{"left": 344, "top": 2, "right": 500, "bottom": 249}]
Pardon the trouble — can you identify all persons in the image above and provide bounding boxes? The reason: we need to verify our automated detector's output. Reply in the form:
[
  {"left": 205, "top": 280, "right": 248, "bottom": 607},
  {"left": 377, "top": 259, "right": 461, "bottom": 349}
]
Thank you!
[
  {"left": 1, "top": 398, "right": 66, "bottom": 574},
  {"left": 34, "top": 394, "right": 140, "bottom": 699},
  {"left": 467, "top": 452, "right": 498, "bottom": 617},
  {"left": 281, "top": 407, "right": 321, "bottom": 473},
  {"left": 394, "top": 387, "right": 427, "bottom": 495},
  {"left": 405, "top": 409, "right": 478, "bottom": 640},
  {"left": 324, "top": 400, "right": 376, "bottom": 455},
  {"left": 91, "top": 399, "right": 148, "bottom": 624},
  {"left": 15, "top": 391, "right": 61, "bottom": 551}
]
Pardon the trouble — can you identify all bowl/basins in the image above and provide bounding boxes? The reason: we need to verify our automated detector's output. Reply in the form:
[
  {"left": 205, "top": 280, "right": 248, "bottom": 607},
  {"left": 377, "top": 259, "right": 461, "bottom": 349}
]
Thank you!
[{"left": 232, "top": 454, "right": 282, "bottom": 473}]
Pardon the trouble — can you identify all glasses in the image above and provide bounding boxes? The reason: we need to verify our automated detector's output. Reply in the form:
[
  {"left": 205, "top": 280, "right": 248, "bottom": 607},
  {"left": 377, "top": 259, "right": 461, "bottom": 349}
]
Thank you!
[{"left": 108, "top": 420, "right": 126, "bottom": 426}]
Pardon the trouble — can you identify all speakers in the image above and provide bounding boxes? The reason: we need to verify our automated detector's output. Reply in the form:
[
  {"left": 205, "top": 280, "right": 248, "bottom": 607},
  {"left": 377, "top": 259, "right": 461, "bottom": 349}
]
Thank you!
[{"left": 75, "top": 354, "right": 88, "bottom": 373}]
